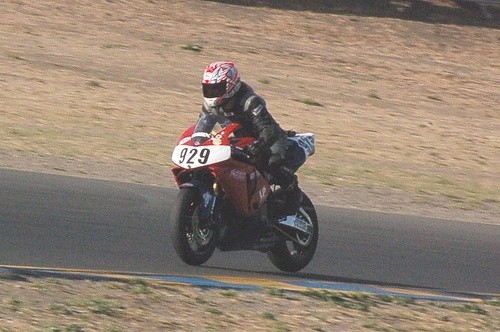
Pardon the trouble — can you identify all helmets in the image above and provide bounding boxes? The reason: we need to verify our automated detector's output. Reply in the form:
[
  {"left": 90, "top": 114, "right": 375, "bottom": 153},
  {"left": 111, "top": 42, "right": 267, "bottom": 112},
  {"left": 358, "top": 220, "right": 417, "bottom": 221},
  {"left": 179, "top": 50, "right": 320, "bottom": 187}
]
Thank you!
[{"left": 201, "top": 61, "right": 242, "bottom": 108}]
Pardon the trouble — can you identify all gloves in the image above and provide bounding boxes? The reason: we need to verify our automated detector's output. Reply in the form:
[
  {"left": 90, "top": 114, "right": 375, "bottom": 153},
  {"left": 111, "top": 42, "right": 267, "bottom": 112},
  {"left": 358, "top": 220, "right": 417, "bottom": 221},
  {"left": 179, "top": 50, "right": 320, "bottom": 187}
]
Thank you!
[{"left": 241, "top": 140, "right": 264, "bottom": 161}]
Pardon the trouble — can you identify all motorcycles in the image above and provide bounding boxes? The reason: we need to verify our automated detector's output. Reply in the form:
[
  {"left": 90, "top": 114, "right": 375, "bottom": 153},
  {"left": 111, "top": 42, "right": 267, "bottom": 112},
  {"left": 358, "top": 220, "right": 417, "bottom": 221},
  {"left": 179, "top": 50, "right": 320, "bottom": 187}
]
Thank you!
[{"left": 169, "top": 95, "right": 320, "bottom": 273}]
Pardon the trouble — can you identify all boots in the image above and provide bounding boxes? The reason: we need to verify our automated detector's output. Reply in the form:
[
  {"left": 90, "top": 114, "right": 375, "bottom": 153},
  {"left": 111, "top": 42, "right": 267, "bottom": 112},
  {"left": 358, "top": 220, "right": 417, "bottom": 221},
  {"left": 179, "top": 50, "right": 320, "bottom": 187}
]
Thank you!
[{"left": 280, "top": 175, "right": 303, "bottom": 215}]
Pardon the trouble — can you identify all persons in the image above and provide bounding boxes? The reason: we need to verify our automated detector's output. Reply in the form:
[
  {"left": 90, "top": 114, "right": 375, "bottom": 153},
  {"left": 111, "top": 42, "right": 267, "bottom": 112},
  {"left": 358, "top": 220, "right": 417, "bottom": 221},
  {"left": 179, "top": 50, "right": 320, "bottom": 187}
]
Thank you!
[{"left": 191, "top": 62, "right": 304, "bottom": 215}]
